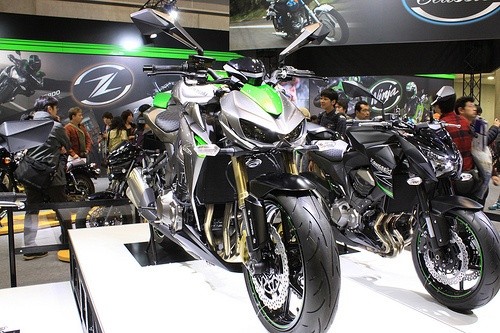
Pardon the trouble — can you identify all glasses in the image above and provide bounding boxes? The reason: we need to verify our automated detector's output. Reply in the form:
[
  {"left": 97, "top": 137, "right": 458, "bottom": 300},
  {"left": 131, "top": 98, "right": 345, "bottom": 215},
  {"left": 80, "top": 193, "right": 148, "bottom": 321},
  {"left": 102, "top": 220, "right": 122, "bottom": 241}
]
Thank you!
[{"left": 361, "top": 109, "right": 372, "bottom": 113}]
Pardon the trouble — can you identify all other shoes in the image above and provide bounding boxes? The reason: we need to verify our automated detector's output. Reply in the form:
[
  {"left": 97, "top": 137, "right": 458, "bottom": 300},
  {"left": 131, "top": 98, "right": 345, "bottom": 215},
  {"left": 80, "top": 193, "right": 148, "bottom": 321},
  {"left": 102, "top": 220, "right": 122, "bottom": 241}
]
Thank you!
[
  {"left": 23, "top": 251, "right": 48, "bottom": 259},
  {"left": 488, "top": 200, "right": 500, "bottom": 210}
]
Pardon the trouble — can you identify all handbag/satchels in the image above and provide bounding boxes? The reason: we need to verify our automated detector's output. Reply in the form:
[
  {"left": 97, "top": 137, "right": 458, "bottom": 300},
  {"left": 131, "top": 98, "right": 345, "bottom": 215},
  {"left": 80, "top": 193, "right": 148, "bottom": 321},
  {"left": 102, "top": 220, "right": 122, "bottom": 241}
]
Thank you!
[
  {"left": 100, "top": 150, "right": 111, "bottom": 167},
  {"left": 12, "top": 155, "right": 56, "bottom": 194}
]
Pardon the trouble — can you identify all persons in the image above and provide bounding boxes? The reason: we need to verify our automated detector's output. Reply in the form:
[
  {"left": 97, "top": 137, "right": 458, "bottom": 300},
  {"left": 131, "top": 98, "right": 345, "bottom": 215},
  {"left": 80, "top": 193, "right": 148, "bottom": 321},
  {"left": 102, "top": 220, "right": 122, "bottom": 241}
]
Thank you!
[
  {"left": 13, "top": 96, "right": 93, "bottom": 259},
  {"left": 354, "top": 101, "right": 370, "bottom": 120},
  {"left": 432, "top": 86, "right": 500, "bottom": 271},
  {"left": 308, "top": 88, "right": 350, "bottom": 177},
  {"left": 97, "top": 104, "right": 152, "bottom": 190}
]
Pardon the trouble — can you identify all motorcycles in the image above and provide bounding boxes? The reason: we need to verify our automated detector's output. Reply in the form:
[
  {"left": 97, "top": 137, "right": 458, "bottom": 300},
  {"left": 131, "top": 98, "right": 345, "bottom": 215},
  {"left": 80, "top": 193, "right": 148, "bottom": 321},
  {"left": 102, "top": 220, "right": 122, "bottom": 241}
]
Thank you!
[
  {"left": 263, "top": 0, "right": 350, "bottom": 46},
  {"left": 0, "top": 145, "right": 100, "bottom": 213},
  {"left": 124, "top": 7, "right": 343, "bottom": 333},
  {"left": 75, "top": 104, "right": 172, "bottom": 229},
  {"left": 1, "top": 51, "right": 46, "bottom": 104},
  {"left": 293, "top": 78, "right": 500, "bottom": 313}
]
